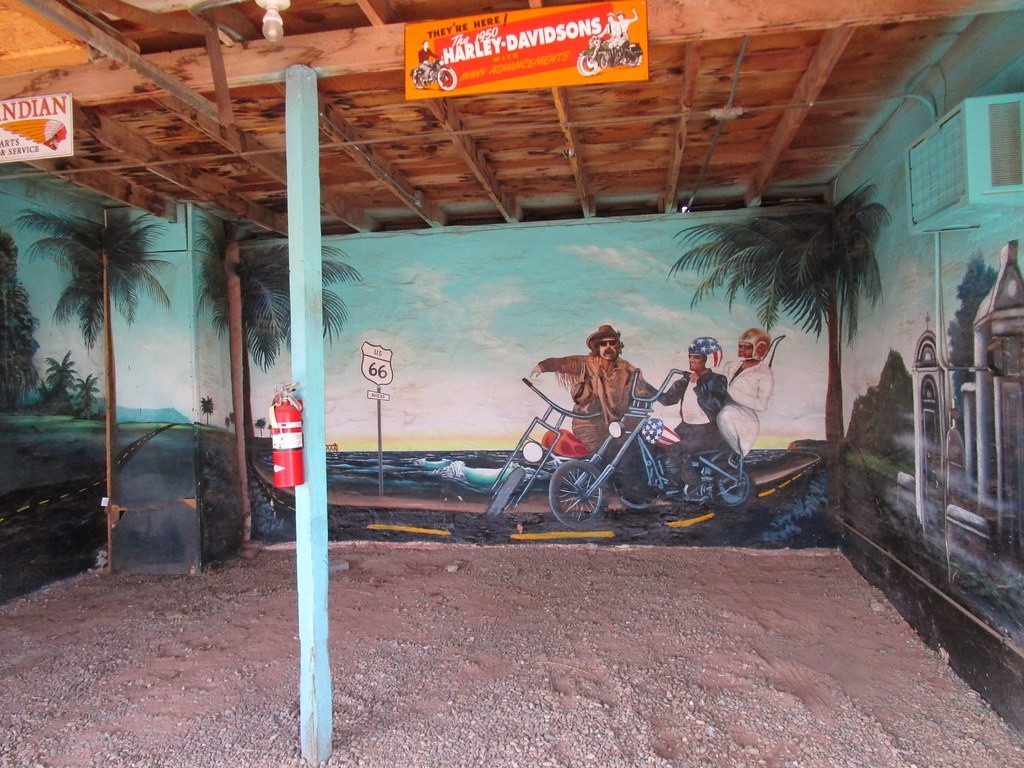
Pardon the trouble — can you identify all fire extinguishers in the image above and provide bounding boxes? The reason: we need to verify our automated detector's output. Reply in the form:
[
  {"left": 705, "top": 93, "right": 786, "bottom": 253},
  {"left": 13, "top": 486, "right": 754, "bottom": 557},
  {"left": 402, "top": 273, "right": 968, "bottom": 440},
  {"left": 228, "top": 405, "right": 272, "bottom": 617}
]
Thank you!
[{"left": 267, "top": 381, "right": 306, "bottom": 487}]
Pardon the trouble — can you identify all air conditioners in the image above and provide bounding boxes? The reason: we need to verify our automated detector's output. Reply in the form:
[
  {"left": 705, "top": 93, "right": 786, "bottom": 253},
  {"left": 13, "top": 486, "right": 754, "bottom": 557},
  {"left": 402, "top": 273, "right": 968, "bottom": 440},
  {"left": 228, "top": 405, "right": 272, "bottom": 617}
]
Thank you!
[{"left": 904, "top": 92, "right": 1024, "bottom": 237}]
